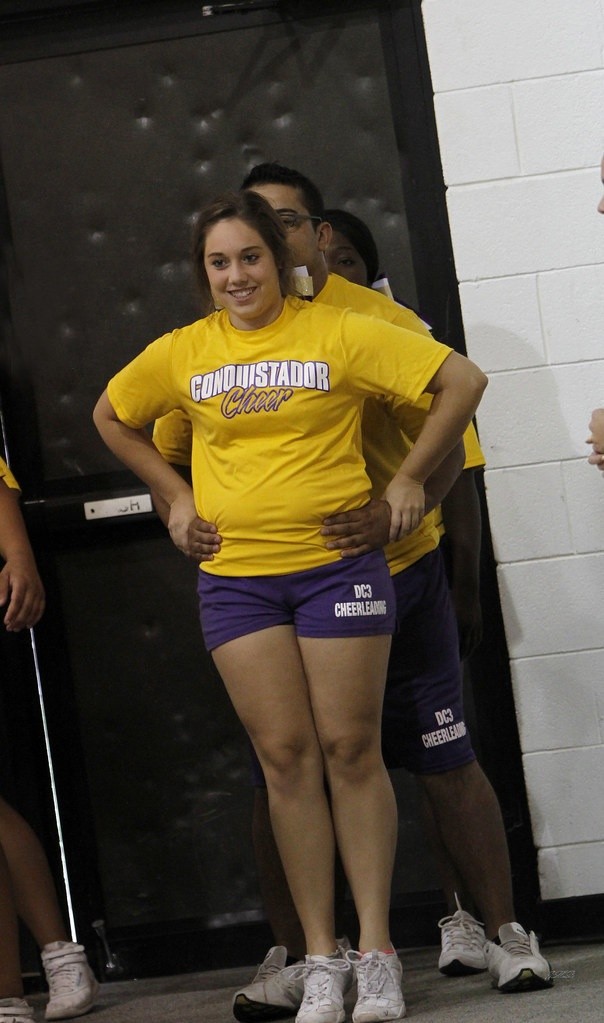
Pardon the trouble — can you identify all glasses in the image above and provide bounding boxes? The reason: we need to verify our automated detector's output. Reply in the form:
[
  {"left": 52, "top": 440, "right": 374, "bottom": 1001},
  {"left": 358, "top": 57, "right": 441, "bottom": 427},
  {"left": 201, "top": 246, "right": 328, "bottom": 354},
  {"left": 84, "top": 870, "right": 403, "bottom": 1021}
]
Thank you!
[{"left": 280, "top": 214, "right": 322, "bottom": 229}]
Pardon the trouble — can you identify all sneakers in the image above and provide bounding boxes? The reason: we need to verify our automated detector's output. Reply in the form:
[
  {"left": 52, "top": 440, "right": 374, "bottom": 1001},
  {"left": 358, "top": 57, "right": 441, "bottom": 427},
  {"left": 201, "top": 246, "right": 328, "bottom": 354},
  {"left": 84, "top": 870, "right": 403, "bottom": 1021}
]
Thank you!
[
  {"left": 345, "top": 942, "right": 405, "bottom": 1023},
  {"left": 232, "top": 961, "right": 304, "bottom": 1023},
  {"left": 0, "top": 997, "right": 34, "bottom": 1023},
  {"left": 40, "top": 941, "right": 99, "bottom": 1020},
  {"left": 437, "top": 891, "right": 488, "bottom": 974},
  {"left": 288, "top": 944, "right": 353, "bottom": 1023},
  {"left": 248, "top": 946, "right": 286, "bottom": 982},
  {"left": 482, "top": 922, "right": 553, "bottom": 991}
]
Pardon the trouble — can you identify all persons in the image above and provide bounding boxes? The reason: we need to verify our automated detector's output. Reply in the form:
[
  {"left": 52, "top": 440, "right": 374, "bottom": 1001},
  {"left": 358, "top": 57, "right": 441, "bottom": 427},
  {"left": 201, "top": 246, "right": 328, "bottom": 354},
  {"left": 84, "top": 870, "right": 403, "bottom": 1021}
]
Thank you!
[
  {"left": 583, "top": 156, "right": 604, "bottom": 477},
  {"left": 230, "top": 207, "right": 493, "bottom": 985},
  {"left": 143, "top": 163, "right": 552, "bottom": 1019},
  {"left": 0, "top": 457, "right": 102, "bottom": 1020},
  {"left": 93, "top": 191, "right": 489, "bottom": 1023}
]
